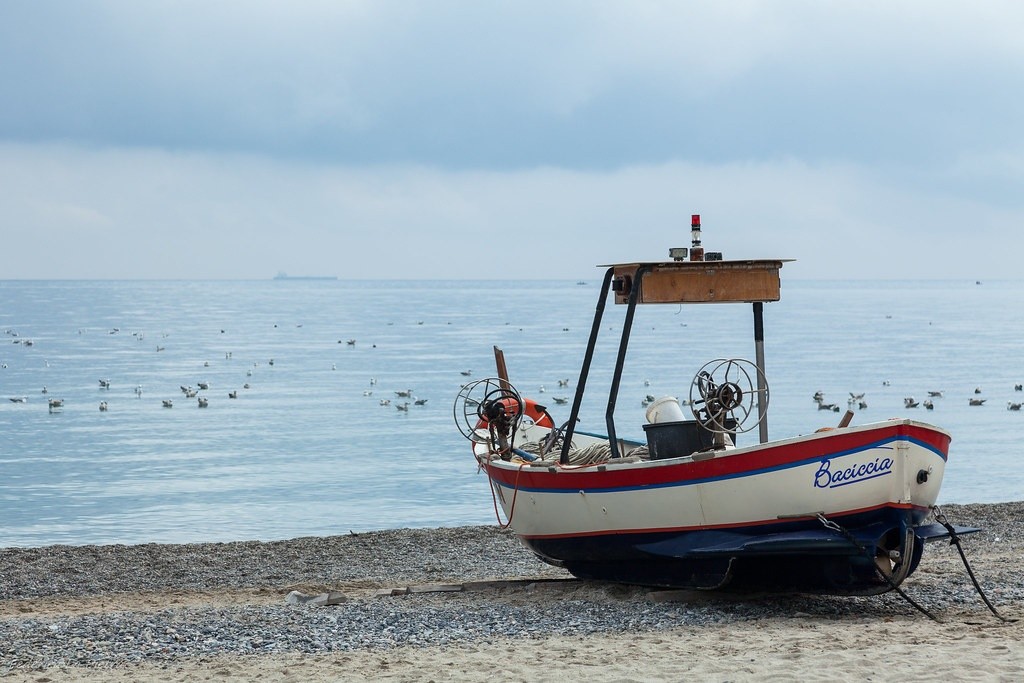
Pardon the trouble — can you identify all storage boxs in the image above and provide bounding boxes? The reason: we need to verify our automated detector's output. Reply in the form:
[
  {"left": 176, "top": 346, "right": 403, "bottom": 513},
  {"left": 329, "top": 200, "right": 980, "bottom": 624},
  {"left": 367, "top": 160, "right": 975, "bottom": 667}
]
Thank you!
[{"left": 643, "top": 417, "right": 739, "bottom": 461}]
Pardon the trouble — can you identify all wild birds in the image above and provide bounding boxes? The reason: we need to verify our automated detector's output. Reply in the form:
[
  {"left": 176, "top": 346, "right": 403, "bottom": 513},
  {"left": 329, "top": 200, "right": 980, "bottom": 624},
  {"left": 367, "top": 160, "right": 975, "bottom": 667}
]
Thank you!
[{"left": 2, "top": 320, "right": 1024, "bottom": 415}]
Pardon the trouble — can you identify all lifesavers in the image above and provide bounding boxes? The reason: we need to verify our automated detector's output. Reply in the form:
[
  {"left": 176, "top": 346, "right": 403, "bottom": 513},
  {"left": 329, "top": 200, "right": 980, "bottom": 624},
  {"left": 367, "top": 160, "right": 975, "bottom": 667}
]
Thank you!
[{"left": 472, "top": 397, "right": 556, "bottom": 466}]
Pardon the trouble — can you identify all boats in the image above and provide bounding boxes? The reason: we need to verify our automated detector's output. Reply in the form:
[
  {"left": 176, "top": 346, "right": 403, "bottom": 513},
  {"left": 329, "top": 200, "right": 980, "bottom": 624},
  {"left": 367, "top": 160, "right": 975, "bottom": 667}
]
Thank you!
[{"left": 449, "top": 215, "right": 983, "bottom": 603}]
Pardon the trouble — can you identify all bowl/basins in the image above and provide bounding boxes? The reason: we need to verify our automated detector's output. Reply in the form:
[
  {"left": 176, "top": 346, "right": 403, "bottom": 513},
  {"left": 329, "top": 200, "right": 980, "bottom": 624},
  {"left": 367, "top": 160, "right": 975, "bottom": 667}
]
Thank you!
[{"left": 641, "top": 417, "right": 740, "bottom": 460}]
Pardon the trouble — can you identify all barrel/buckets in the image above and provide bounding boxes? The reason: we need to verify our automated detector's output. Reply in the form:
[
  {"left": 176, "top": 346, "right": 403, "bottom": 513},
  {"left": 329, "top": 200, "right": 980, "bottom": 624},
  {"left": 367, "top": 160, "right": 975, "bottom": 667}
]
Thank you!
[{"left": 645, "top": 395, "right": 685, "bottom": 424}]
